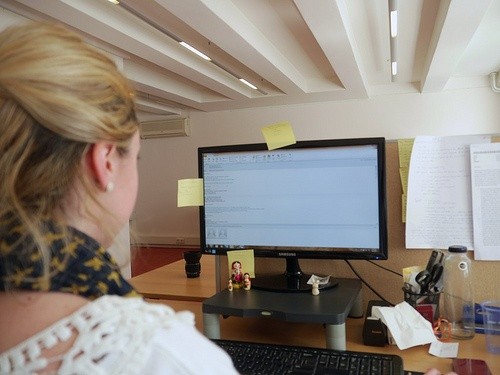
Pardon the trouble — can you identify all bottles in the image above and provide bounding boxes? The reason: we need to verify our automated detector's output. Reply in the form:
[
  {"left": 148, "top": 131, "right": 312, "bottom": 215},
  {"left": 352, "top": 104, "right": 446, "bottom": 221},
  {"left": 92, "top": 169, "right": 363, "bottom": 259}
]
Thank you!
[{"left": 442, "top": 245, "right": 476, "bottom": 340}]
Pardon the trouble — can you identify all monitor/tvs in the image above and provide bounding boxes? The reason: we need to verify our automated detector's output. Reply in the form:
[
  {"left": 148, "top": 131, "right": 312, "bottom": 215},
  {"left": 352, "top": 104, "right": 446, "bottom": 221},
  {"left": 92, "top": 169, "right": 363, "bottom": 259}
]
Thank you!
[{"left": 198, "top": 137, "right": 389, "bottom": 292}]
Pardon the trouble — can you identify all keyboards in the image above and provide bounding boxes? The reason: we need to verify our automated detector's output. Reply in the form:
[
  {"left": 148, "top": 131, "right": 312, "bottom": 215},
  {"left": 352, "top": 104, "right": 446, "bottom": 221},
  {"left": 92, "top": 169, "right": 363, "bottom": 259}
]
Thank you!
[{"left": 211, "top": 338, "right": 405, "bottom": 375}]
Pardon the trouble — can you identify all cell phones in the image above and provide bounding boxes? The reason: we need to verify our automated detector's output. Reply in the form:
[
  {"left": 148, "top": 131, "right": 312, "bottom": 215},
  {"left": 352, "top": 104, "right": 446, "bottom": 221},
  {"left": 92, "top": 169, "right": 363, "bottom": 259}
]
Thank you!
[{"left": 452, "top": 358, "right": 492, "bottom": 375}]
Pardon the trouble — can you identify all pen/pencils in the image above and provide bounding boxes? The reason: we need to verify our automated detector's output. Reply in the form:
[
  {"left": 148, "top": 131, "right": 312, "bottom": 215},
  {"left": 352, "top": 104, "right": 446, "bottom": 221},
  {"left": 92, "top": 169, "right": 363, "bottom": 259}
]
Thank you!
[{"left": 416, "top": 286, "right": 438, "bottom": 304}]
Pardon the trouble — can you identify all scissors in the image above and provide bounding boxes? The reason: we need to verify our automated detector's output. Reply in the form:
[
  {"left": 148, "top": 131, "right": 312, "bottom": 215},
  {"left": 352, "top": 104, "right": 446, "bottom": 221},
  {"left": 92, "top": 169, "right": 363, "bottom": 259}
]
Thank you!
[{"left": 415, "top": 264, "right": 443, "bottom": 295}]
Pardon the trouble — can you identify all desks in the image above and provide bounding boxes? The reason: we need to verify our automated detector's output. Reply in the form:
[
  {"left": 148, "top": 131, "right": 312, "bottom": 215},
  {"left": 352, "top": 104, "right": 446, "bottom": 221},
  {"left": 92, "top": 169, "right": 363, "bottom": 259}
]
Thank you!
[
  {"left": 167, "top": 304, "right": 500, "bottom": 375},
  {"left": 124, "top": 253, "right": 215, "bottom": 304}
]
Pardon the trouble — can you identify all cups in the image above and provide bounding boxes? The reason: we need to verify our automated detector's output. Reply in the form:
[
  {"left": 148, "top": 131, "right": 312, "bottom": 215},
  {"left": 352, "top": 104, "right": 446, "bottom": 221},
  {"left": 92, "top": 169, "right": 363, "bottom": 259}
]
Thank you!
[{"left": 481, "top": 299, "right": 500, "bottom": 352}]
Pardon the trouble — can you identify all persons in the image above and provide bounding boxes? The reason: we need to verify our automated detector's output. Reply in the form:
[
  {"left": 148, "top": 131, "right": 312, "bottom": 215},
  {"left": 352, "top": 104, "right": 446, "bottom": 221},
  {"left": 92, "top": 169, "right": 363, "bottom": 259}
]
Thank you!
[
  {"left": 0, "top": 24, "right": 241, "bottom": 374},
  {"left": 229, "top": 261, "right": 250, "bottom": 289}
]
